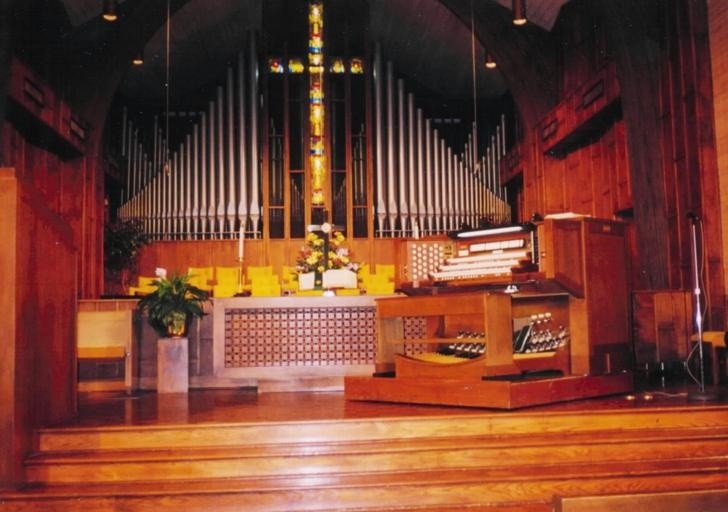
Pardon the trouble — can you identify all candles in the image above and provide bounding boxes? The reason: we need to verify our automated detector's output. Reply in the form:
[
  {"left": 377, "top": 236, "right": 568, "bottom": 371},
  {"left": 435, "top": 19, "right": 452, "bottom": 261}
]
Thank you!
[{"left": 238, "top": 225, "right": 244, "bottom": 259}]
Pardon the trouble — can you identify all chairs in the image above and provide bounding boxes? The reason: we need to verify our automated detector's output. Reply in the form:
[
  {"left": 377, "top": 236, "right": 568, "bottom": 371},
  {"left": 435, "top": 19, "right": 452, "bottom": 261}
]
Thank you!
[{"left": 137, "top": 265, "right": 396, "bottom": 296}]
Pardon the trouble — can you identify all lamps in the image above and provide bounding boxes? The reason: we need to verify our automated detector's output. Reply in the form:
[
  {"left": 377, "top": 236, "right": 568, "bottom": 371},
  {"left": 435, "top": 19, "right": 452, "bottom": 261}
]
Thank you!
[
  {"left": 133, "top": 52, "right": 143, "bottom": 64},
  {"left": 485, "top": 53, "right": 496, "bottom": 68},
  {"left": 101, "top": 0, "right": 118, "bottom": 21},
  {"left": 513, "top": 0, "right": 527, "bottom": 25}
]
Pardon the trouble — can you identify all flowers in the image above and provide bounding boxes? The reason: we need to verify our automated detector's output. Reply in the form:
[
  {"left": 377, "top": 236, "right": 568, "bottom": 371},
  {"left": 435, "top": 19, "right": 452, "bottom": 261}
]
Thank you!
[{"left": 291, "top": 232, "right": 359, "bottom": 285}]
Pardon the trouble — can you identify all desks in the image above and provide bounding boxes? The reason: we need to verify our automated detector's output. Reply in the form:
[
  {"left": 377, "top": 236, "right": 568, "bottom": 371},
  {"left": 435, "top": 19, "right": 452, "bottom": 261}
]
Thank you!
[{"left": 375, "top": 290, "right": 566, "bottom": 377}]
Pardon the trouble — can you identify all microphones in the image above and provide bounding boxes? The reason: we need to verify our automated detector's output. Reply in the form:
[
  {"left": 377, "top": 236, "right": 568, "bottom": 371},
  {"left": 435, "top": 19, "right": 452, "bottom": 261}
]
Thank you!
[{"left": 685, "top": 212, "right": 699, "bottom": 224}]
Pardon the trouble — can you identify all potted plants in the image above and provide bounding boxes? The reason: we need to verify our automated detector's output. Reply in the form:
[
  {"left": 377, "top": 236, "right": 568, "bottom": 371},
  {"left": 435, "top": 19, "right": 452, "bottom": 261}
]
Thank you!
[
  {"left": 104, "top": 211, "right": 153, "bottom": 297},
  {"left": 137, "top": 276, "right": 212, "bottom": 337}
]
